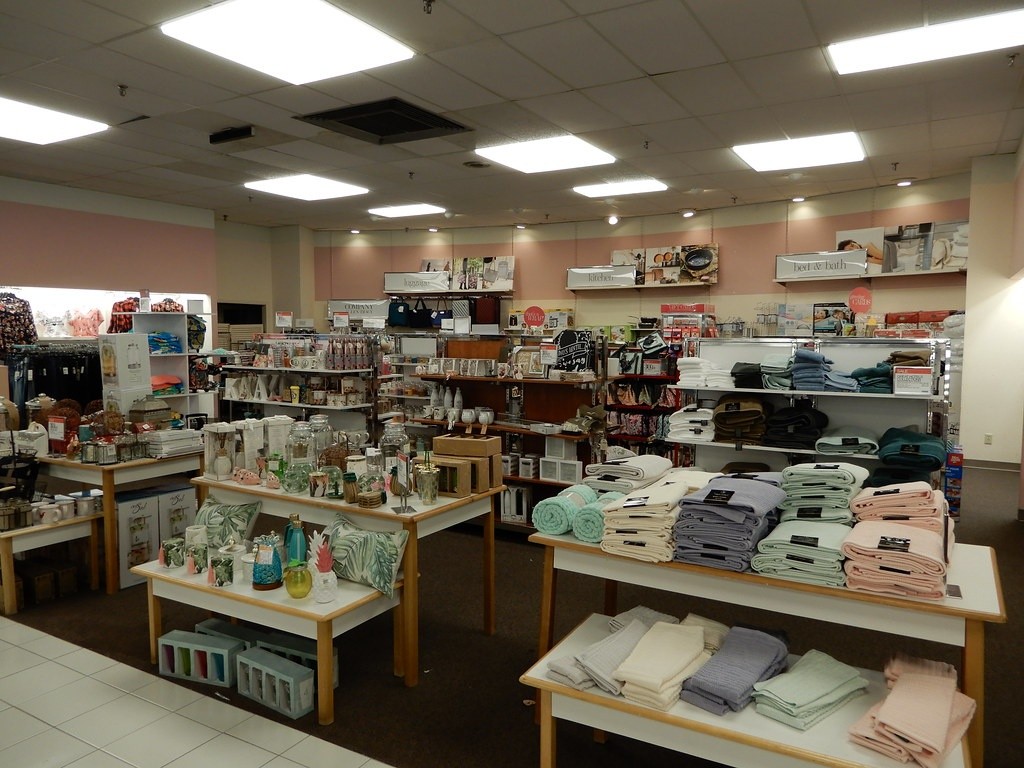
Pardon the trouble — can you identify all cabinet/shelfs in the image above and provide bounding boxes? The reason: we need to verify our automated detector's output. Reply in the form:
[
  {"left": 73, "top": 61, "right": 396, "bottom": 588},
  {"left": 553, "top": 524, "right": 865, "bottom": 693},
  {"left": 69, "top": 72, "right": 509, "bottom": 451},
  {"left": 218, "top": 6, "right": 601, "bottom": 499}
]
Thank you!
[
  {"left": 112, "top": 312, "right": 241, "bottom": 430},
  {"left": 382, "top": 289, "right": 513, "bottom": 336},
  {"left": 664, "top": 336, "right": 953, "bottom": 491},
  {"left": 604, "top": 373, "right": 696, "bottom": 469},
  {"left": 407, "top": 366, "right": 604, "bottom": 537},
  {"left": 220, "top": 365, "right": 376, "bottom": 452}
]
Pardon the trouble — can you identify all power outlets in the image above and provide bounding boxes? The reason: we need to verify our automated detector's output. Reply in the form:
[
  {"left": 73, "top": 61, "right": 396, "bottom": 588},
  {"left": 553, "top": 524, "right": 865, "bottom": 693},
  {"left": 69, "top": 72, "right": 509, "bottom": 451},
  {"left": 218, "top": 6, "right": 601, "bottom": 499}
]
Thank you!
[{"left": 985, "top": 434, "right": 992, "bottom": 445}]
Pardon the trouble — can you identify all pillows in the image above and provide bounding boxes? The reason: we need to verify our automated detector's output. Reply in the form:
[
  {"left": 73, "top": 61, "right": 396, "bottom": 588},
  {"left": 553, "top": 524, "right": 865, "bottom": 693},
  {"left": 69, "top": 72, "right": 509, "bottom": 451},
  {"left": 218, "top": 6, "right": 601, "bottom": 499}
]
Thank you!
[
  {"left": 310, "top": 512, "right": 408, "bottom": 599},
  {"left": 194, "top": 492, "right": 262, "bottom": 555}
]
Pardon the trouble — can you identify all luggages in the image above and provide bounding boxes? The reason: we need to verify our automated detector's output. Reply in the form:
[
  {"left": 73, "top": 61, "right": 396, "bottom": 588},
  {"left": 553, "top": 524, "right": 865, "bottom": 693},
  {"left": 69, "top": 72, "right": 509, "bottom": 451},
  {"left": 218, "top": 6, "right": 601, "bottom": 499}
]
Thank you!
[
  {"left": 451, "top": 294, "right": 475, "bottom": 325},
  {"left": 497, "top": 261, "right": 509, "bottom": 281},
  {"left": 468, "top": 266, "right": 478, "bottom": 289},
  {"left": 475, "top": 293, "right": 500, "bottom": 324}
]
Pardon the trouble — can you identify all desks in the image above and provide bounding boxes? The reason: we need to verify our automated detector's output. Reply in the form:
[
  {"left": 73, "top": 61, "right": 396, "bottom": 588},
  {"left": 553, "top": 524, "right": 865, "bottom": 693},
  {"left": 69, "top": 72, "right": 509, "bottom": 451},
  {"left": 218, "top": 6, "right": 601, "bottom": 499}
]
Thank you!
[
  {"left": 0, "top": 510, "right": 103, "bottom": 616},
  {"left": 35, "top": 454, "right": 204, "bottom": 597},
  {"left": 129, "top": 546, "right": 421, "bottom": 727},
  {"left": 519, "top": 611, "right": 971, "bottom": 768},
  {"left": 191, "top": 474, "right": 506, "bottom": 678},
  {"left": 527, "top": 530, "right": 1007, "bottom": 768}
]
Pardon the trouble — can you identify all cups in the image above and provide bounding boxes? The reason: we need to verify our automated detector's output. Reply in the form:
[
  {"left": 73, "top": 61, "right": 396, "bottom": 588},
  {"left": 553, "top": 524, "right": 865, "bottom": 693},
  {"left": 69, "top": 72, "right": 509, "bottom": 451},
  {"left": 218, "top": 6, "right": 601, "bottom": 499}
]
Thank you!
[
  {"left": 311, "top": 390, "right": 364, "bottom": 407},
  {"left": 513, "top": 363, "right": 524, "bottom": 380},
  {"left": 497, "top": 363, "right": 511, "bottom": 379},
  {"left": 289, "top": 350, "right": 327, "bottom": 369},
  {"left": 290, "top": 385, "right": 300, "bottom": 404},
  {"left": 308, "top": 472, "right": 328, "bottom": 497},
  {"left": 30, "top": 497, "right": 95, "bottom": 524},
  {"left": 415, "top": 463, "right": 440, "bottom": 506},
  {"left": 423, "top": 405, "right": 494, "bottom": 425},
  {"left": 345, "top": 454, "right": 367, "bottom": 480}
]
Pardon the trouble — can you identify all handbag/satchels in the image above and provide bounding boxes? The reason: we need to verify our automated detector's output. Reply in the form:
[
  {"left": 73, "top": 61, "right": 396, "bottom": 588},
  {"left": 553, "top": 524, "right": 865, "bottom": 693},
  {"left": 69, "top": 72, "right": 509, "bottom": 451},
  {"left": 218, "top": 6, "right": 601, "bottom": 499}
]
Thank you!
[
  {"left": 429, "top": 296, "right": 452, "bottom": 325},
  {"left": 458, "top": 273, "right": 465, "bottom": 283},
  {"left": 484, "top": 260, "right": 498, "bottom": 283},
  {"left": 409, "top": 296, "right": 432, "bottom": 328},
  {"left": 388, "top": 295, "right": 409, "bottom": 326}
]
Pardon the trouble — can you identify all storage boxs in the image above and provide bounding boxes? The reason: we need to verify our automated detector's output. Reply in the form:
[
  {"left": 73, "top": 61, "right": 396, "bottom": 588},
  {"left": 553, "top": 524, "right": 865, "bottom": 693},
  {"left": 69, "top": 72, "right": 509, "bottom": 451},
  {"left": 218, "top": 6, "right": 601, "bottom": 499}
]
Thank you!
[
  {"left": 115, "top": 481, "right": 199, "bottom": 587},
  {"left": 944, "top": 448, "right": 963, "bottom": 522},
  {"left": 507, "top": 307, "right": 637, "bottom": 344},
  {"left": 97, "top": 332, "right": 154, "bottom": 423},
  {"left": 755, "top": 301, "right": 957, "bottom": 341},
  {"left": 500, "top": 482, "right": 532, "bottom": 524}
]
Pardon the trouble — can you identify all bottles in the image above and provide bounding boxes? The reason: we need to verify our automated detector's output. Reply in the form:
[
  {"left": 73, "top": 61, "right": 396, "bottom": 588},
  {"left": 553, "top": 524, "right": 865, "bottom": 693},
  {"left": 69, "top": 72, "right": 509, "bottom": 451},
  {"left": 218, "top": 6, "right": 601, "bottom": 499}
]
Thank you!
[
  {"left": 380, "top": 422, "right": 410, "bottom": 474},
  {"left": 285, "top": 522, "right": 306, "bottom": 565},
  {"left": 286, "top": 414, "right": 334, "bottom": 470},
  {"left": 127, "top": 344, "right": 143, "bottom": 385},
  {"left": 367, "top": 448, "right": 385, "bottom": 477},
  {"left": 430, "top": 387, "right": 463, "bottom": 409},
  {"left": 253, "top": 535, "right": 282, "bottom": 590},
  {"left": 380, "top": 378, "right": 432, "bottom": 424},
  {"left": 101, "top": 340, "right": 119, "bottom": 385}
]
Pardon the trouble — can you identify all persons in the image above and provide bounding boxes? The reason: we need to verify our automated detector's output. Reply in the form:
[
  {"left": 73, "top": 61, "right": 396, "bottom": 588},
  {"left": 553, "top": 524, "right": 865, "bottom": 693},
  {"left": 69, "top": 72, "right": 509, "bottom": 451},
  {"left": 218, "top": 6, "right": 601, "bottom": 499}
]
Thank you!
[
  {"left": 815, "top": 309, "right": 829, "bottom": 319},
  {"left": 832, "top": 309, "right": 845, "bottom": 319},
  {"left": 837, "top": 239, "right": 884, "bottom": 265}
]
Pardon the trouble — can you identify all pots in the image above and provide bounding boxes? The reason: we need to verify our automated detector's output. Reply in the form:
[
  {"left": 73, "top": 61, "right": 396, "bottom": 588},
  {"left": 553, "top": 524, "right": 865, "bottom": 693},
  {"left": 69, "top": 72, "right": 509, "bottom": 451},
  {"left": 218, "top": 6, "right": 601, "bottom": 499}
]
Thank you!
[{"left": 684, "top": 249, "right": 714, "bottom": 270}]
